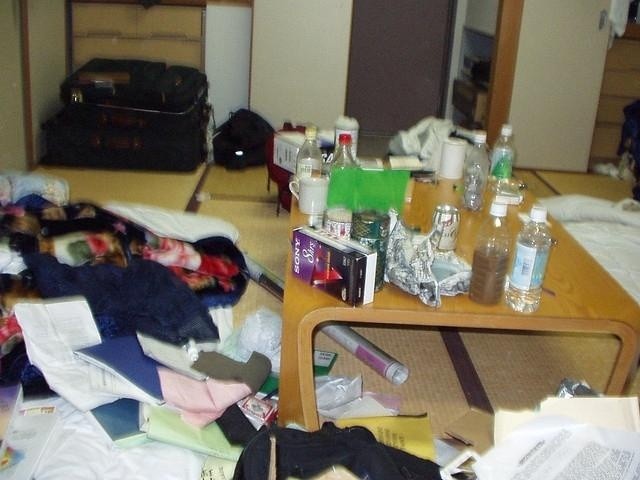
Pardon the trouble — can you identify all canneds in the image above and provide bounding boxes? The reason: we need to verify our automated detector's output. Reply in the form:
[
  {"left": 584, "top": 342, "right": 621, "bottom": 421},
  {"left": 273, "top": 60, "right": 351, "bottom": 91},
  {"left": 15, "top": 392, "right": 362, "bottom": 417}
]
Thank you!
[
  {"left": 326, "top": 208, "right": 353, "bottom": 242},
  {"left": 430, "top": 204, "right": 462, "bottom": 250}
]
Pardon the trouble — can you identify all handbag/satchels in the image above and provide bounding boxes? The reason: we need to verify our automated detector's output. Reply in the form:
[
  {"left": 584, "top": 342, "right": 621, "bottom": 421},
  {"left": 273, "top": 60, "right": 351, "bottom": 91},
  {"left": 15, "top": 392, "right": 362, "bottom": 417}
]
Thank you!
[
  {"left": 213, "top": 109, "right": 274, "bottom": 170},
  {"left": 233, "top": 422, "right": 467, "bottom": 480}
]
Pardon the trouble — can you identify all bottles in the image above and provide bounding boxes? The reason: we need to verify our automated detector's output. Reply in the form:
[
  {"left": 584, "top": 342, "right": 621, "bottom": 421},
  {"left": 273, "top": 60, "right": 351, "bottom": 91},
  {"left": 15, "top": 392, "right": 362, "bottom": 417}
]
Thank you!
[
  {"left": 506, "top": 207, "right": 552, "bottom": 313},
  {"left": 461, "top": 129, "right": 489, "bottom": 211},
  {"left": 489, "top": 124, "right": 517, "bottom": 193},
  {"left": 468, "top": 202, "right": 511, "bottom": 307},
  {"left": 352, "top": 209, "right": 391, "bottom": 293},
  {"left": 295, "top": 126, "right": 322, "bottom": 194},
  {"left": 327, "top": 134, "right": 356, "bottom": 180}
]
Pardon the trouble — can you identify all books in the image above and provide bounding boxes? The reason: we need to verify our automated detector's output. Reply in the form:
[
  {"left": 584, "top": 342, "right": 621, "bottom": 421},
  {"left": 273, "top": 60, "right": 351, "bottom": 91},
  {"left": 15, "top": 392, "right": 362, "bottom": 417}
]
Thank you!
[
  {"left": 89, "top": 395, "right": 148, "bottom": 444},
  {"left": 0, "top": 410, "right": 62, "bottom": 480},
  {"left": 25, "top": 404, "right": 58, "bottom": 414},
  {"left": 312, "top": 349, "right": 340, "bottom": 379},
  {"left": 255, "top": 368, "right": 280, "bottom": 402},
  {"left": 233, "top": 396, "right": 280, "bottom": 431},
  {"left": 1, "top": 382, "right": 26, "bottom": 462},
  {"left": 74, "top": 333, "right": 168, "bottom": 408}
]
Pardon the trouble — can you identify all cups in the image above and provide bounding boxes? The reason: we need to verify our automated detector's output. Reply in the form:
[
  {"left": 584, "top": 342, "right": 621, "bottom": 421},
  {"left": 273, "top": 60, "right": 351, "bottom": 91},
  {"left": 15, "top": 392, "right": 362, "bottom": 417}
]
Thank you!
[
  {"left": 289, "top": 177, "right": 329, "bottom": 215},
  {"left": 323, "top": 208, "right": 353, "bottom": 240},
  {"left": 439, "top": 137, "right": 467, "bottom": 180}
]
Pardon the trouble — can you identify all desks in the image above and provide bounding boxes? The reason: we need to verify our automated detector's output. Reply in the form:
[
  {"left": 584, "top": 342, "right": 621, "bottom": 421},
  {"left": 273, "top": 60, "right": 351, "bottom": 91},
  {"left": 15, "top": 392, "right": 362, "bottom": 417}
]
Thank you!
[{"left": 279, "top": 163, "right": 640, "bottom": 432}]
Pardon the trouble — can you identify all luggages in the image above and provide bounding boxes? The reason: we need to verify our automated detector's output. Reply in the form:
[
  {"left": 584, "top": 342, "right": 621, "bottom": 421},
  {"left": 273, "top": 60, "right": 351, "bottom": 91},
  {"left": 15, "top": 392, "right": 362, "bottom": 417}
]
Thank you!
[{"left": 39, "top": 58, "right": 209, "bottom": 172}]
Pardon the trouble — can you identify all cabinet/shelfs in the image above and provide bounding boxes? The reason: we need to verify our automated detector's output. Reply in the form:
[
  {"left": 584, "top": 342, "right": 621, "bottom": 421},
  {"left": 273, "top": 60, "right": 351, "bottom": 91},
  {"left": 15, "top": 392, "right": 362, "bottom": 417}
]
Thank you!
[{"left": 68, "top": 0, "right": 253, "bottom": 131}]
